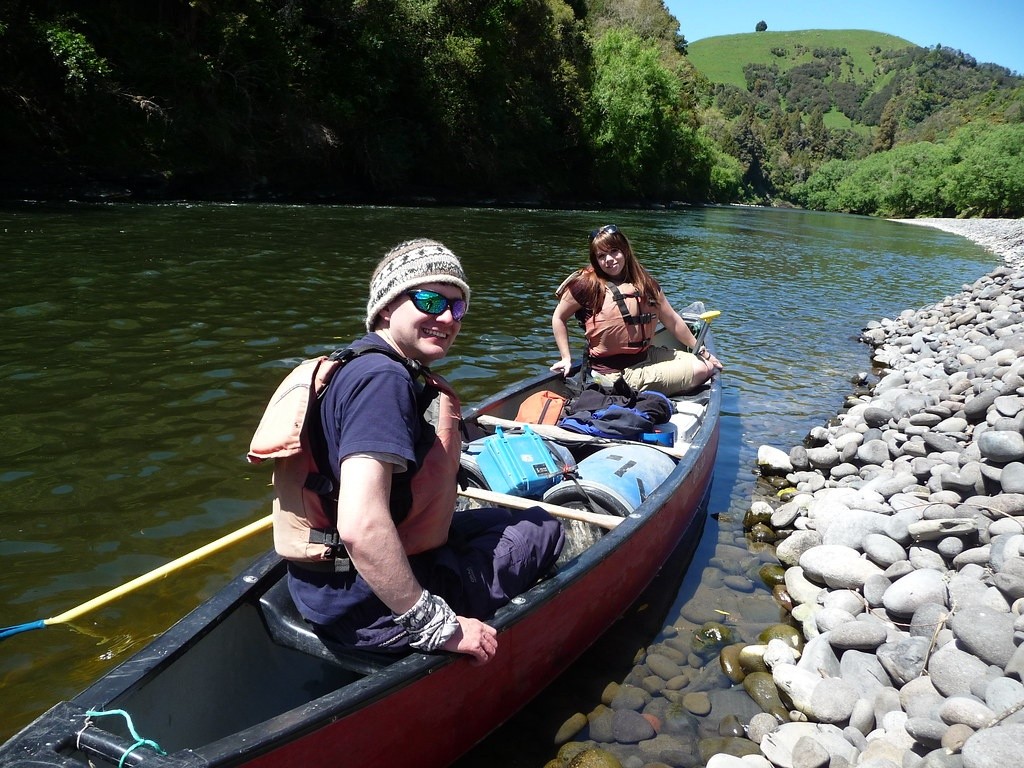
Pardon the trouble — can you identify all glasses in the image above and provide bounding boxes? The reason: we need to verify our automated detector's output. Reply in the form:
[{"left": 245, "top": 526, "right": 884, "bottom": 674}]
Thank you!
[
  {"left": 588, "top": 225, "right": 623, "bottom": 244},
  {"left": 402, "top": 288, "right": 466, "bottom": 322}
]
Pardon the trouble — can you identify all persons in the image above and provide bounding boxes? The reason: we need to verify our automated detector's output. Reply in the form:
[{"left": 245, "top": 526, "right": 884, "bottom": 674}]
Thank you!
[
  {"left": 247, "top": 238, "right": 566, "bottom": 667},
  {"left": 550, "top": 226, "right": 724, "bottom": 396}
]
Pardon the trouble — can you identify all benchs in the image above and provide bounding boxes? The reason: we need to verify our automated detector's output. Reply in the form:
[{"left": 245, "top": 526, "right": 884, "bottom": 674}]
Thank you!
[
  {"left": 560, "top": 371, "right": 711, "bottom": 405},
  {"left": 251, "top": 572, "right": 412, "bottom": 676}
]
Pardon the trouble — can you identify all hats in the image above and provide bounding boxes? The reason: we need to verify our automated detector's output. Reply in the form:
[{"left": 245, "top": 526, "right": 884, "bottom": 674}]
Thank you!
[{"left": 365, "top": 238, "right": 470, "bottom": 334}]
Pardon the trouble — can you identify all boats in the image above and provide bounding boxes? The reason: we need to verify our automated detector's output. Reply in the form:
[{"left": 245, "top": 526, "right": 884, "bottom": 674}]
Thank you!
[{"left": 0, "top": 302, "right": 724, "bottom": 761}]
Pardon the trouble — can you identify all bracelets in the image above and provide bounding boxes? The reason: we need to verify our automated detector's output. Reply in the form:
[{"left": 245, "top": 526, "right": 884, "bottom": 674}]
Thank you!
[
  {"left": 392, "top": 589, "right": 460, "bottom": 653},
  {"left": 706, "top": 353, "right": 711, "bottom": 360},
  {"left": 698, "top": 346, "right": 706, "bottom": 353}
]
